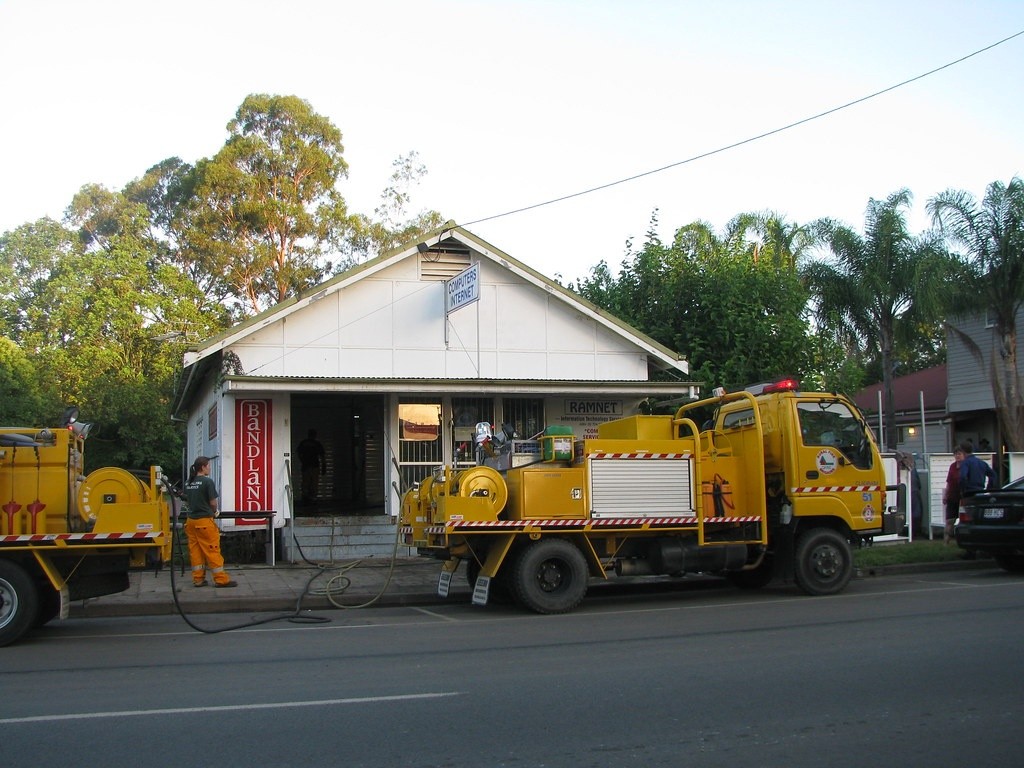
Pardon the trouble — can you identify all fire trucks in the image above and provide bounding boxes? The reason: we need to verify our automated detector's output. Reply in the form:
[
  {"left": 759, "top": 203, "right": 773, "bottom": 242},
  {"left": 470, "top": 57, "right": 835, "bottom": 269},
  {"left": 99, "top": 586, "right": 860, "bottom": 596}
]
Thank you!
[
  {"left": 0, "top": 406, "right": 173, "bottom": 647},
  {"left": 392, "top": 379, "right": 909, "bottom": 615}
]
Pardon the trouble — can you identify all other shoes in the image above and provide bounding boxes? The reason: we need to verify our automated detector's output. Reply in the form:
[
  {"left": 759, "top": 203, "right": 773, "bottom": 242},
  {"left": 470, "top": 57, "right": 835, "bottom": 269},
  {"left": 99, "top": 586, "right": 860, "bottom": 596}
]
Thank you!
[
  {"left": 195, "top": 580, "right": 208, "bottom": 588},
  {"left": 216, "top": 580, "right": 237, "bottom": 588}
]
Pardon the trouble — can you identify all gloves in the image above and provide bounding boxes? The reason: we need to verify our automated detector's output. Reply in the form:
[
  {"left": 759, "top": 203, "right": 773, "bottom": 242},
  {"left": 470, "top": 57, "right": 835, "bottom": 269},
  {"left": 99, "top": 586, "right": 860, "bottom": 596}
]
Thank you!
[{"left": 215, "top": 509, "right": 220, "bottom": 518}]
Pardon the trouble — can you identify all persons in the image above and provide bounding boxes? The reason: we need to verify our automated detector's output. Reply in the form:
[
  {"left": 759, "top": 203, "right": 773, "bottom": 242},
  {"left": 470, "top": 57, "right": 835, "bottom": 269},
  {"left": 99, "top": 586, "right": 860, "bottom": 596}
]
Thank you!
[
  {"left": 958, "top": 441, "right": 997, "bottom": 492},
  {"left": 965, "top": 438, "right": 978, "bottom": 452},
  {"left": 296, "top": 429, "right": 326, "bottom": 505},
  {"left": 942, "top": 446, "right": 965, "bottom": 544},
  {"left": 978, "top": 438, "right": 993, "bottom": 451},
  {"left": 184, "top": 457, "right": 237, "bottom": 588}
]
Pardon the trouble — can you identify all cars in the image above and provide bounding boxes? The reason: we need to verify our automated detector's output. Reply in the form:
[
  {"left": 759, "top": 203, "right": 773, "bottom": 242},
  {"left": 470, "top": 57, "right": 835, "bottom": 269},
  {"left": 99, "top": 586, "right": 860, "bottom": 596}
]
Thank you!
[{"left": 954, "top": 475, "right": 1024, "bottom": 573}]
